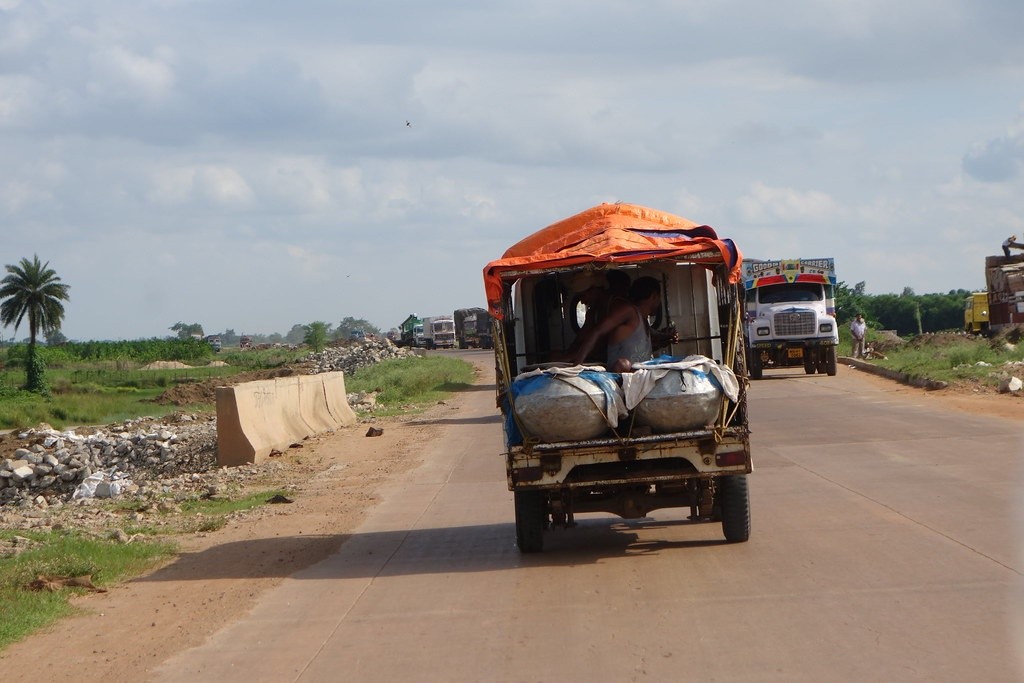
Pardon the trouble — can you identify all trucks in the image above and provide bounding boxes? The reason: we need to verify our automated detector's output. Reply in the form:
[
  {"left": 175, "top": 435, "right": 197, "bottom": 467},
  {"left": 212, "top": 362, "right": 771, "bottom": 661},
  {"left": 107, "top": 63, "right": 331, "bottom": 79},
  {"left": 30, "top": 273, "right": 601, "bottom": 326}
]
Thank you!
[
  {"left": 454, "top": 307, "right": 485, "bottom": 350},
  {"left": 421, "top": 316, "right": 456, "bottom": 351},
  {"left": 483, "top": 201, "right": 754, "bottom": 558},
  {"left": 964, "top": 292, "right": 992, "bottom": 337},
  {"left": 399, "top": 313, "right": 426, "bottom": 347},
  {"left": 479, "top": 311, "right": 493, "bottom": 350},
  {"left": 738, "top": 256, "right": 839, "bottom": 379}
]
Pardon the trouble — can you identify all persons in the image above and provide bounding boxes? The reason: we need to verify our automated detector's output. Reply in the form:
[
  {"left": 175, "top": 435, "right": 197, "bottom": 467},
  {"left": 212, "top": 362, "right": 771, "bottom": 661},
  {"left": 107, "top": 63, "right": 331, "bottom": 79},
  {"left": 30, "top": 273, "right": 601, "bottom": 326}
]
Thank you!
[
  {"left": 850, "top": 313, "right": 867, "bottom": 357},
  {"left": 545, "top": 284, "right": 625, "bottom": 362},
  {"left": 607, "top": 268, "right": 626, "bottom": 291},
  {"left": 571, "top": 275, "right": 679, "bottom": 373},
  {"left": 1002, "top": 235, "right": 1016, "bottom": 257}
]
